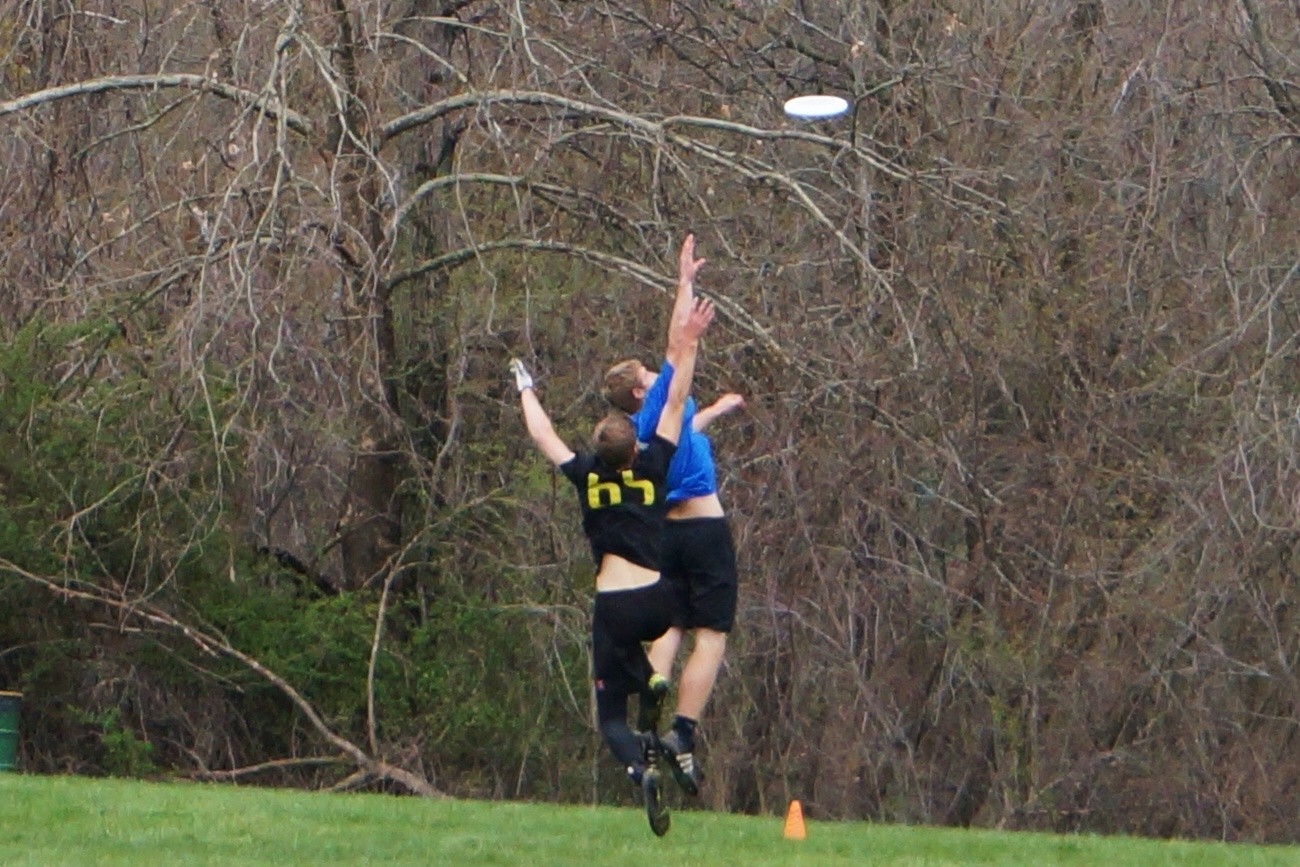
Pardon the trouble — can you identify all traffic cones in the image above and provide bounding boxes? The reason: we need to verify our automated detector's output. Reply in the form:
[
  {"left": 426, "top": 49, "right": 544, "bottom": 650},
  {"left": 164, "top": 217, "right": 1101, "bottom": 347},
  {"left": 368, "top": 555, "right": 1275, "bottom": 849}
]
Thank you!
[{"left": 783, "top": 800, "right": 808, "bottom": 841}]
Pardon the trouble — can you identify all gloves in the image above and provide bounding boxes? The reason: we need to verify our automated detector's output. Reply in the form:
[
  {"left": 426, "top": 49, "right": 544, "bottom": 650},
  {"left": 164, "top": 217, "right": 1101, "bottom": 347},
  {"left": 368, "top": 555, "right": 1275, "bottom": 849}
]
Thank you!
[{"left": 509, "top": 358, "right": 533, "bottom": 391}]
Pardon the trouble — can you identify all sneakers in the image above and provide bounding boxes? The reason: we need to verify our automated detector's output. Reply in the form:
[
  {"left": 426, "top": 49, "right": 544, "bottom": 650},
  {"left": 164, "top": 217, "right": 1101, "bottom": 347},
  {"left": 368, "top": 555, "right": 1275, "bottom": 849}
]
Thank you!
[
  {"left": 637, "top": 675, "right": 667, "bottom": 734},
  {"left": 642, "top": 770, "right": 670, "bottom": 837},
  {"left": 658, "top": 730, "right": 699, "bottom": 798}
]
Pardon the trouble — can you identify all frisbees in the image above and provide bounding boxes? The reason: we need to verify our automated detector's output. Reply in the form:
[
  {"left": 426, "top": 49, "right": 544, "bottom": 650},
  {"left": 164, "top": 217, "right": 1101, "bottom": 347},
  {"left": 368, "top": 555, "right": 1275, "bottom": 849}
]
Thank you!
[{"left": 784, "top": 95, "right": 848, "bottom": 117}]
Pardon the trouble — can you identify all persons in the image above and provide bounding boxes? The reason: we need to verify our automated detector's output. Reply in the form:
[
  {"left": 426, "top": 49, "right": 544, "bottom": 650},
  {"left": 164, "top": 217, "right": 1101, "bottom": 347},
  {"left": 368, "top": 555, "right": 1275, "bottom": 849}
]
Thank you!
[
  {"left": 508, "top": 296, "right": 715, "bottom": 838},
  {"left": 601, "top": 232, "right": 745, "bottom": 794}
]
